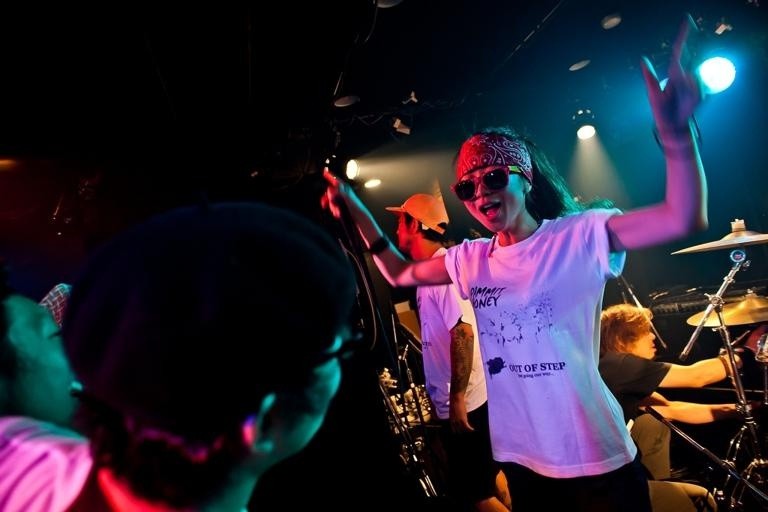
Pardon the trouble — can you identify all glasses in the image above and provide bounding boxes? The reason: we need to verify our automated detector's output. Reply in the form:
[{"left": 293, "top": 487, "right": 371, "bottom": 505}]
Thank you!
[
  {"left": 450, "top": 169, "right": 522, "bottom": 200},
  {"left": 327, "top": 324, "right": 371, "bottom": 361}
]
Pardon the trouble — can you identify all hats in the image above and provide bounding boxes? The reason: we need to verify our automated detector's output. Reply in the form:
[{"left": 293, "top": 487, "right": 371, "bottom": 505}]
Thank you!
[
  {"left": 385, "top": 193, "right": 449, "bottom": 235},
  {"left": 60, "top": 202, "right": 355, "bottom": 421}
]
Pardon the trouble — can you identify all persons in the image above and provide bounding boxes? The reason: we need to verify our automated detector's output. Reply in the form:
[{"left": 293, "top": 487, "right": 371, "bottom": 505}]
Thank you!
[
  {"left": 320, "top": 25, "right": 768, "bottom": 511},
  {"left": 1, "top": 200, "right": 359, "bottom": 509}
]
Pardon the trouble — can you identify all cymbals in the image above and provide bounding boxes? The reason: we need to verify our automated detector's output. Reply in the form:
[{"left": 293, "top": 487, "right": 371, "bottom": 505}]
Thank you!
[
  {"left": 686, "top": 296, "right": 768, "bottom": 327},
  {"left": 671, "top": 231, "right": 768, "bottom": 255}
]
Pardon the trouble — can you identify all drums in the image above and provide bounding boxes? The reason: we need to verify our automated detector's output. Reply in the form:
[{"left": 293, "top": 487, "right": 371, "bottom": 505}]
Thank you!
[{"left": 383, "top": 385, "right": 432, "bottom": 433}]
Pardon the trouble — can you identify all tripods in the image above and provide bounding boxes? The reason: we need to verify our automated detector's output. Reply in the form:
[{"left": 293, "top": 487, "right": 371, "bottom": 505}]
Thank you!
[{"left": 713, "top": 304, "right": 768, "bottom": 512}]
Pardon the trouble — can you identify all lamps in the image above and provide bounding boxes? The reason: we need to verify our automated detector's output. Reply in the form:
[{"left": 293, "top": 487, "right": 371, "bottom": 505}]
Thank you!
[
  {"left": 571, "top": 103, "right": 596, "bottom": 141},
  {"left": 319, "top": 154, "right": 360, "bottom": 180}
]
